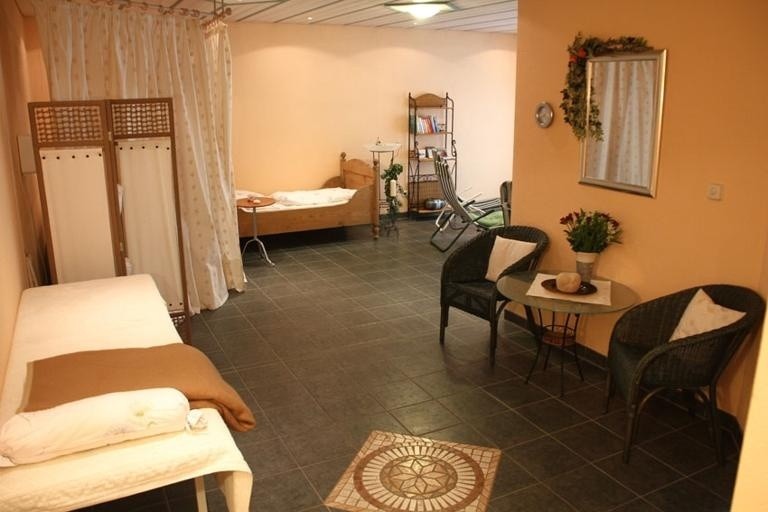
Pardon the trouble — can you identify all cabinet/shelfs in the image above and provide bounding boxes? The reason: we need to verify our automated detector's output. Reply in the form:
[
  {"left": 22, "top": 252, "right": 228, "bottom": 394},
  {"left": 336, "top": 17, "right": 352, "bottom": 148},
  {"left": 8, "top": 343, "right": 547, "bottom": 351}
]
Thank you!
[{"left": 409, "top": 92, "right": 457, "bottom": 219}]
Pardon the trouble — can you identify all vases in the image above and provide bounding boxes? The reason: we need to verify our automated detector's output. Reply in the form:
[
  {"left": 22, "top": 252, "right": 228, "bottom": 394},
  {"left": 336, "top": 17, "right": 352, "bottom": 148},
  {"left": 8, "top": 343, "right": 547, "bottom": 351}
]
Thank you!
[{"left": 576, "top": 252, "right": 597, "bottom": 281}]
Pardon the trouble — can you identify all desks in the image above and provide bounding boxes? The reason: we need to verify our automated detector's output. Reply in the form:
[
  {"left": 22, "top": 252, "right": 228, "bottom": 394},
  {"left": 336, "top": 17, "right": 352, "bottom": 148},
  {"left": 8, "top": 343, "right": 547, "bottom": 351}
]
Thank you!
[
  {"left": 496, "top": 271, "right": 636, "bottom": 398},
  {"left": 236, "top": 197, "right": 276, "bottom": 266}
]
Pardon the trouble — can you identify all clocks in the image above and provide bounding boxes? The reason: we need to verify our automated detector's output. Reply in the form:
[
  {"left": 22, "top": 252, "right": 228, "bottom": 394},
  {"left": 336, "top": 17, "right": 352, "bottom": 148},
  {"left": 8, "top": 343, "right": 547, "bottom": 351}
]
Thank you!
[{"left": 535, "top": 102, "right": 554, "bottom": 128}]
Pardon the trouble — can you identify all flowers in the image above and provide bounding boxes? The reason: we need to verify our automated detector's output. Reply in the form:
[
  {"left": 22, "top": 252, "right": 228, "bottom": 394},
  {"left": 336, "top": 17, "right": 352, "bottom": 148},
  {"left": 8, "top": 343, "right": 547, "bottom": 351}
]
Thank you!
[
  {"left": 559, "top": 32, "right": 653, "bottom": 140},
  {"left": 560, "top": 208, "right": 623, "bottom": 253}
]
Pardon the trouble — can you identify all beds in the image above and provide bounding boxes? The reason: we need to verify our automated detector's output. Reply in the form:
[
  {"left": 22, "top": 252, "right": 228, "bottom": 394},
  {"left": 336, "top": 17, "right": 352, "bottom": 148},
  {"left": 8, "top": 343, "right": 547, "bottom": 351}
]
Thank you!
[
  {"left": 235, "top": 152, "right": 380, "bottom": 239},
  {"left": 1, "top": 273, "right": 254, "bottom": 512}
]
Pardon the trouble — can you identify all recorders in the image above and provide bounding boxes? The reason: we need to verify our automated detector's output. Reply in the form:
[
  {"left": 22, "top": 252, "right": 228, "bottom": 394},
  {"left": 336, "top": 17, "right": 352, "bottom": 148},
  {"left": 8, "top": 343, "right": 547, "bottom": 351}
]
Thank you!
[{"left": 423, "top": 197, "right": 447, "bottom": 210}]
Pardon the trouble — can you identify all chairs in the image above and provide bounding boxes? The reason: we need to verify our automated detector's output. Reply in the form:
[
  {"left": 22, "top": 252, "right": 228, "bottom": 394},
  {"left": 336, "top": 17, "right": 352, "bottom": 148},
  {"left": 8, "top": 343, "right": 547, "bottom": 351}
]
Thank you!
[
  {"left": 499, "top": 181, "right": 512, "bottom": 227},
  {"left": 429, "top": 148, "right": 502, "bottom": 252},
  {"left": 439, "top": 226, "right": 549, "bottom": 366},
  {"left": 604, "top": 283, "right": 766, "bottom": 463}
]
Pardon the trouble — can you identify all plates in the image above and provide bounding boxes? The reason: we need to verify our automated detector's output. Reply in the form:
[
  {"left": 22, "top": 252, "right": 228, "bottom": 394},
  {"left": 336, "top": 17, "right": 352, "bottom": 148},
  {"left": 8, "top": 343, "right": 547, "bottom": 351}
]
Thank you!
[{"left": 539, "top": 277, "right": 597, "bottom": 296}]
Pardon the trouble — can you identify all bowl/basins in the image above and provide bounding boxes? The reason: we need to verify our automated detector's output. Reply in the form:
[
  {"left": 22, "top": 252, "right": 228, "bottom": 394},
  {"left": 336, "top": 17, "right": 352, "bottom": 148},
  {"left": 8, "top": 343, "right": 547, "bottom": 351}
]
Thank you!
[
  {"left": 246, "top": 193, "right": 257, "bottom": 202},
  {"left": 556, "top": 272, "right": 582, "bottom": 293}
]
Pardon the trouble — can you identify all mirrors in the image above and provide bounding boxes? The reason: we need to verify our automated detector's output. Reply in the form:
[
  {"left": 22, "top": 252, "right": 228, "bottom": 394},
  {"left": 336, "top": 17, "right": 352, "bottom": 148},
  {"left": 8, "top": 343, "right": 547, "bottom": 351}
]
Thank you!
[{"left": 577, "top": 48, "right": 667, "bottom": 199}]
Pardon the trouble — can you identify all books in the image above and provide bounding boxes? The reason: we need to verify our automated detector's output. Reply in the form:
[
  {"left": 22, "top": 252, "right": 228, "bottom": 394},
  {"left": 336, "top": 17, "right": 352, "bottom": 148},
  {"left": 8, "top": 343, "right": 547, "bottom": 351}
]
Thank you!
[{"left": 409, "top": 115, "right": 440, "bottom": 135}]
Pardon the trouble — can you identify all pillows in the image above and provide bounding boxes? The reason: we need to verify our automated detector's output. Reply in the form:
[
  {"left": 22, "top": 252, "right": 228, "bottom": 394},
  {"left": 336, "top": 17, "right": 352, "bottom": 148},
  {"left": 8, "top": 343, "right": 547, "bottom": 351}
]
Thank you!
[
  {"left": 485, "top": 233, "right": 538, "bottom": 282},
  {"left": 0, "top": 387, "right": 190, "bottom": 467},
  {"left": 669, "top": 287, "right": 746, "bottom": 342}
]
